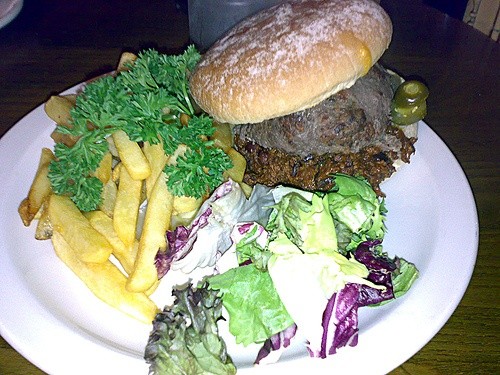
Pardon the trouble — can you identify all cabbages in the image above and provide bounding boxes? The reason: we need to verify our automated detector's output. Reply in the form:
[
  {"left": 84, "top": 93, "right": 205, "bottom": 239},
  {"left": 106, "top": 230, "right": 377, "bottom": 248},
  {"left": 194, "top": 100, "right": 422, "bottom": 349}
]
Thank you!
[{"left": 145, "top": 171, "right": 417, "bottom": 375}]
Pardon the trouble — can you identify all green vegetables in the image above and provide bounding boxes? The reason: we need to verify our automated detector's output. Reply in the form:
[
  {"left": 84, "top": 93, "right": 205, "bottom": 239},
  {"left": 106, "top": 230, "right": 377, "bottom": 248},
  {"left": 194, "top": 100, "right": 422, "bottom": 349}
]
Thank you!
[{"left": 48, "top": 46, "right": 234, "bottom": 210}]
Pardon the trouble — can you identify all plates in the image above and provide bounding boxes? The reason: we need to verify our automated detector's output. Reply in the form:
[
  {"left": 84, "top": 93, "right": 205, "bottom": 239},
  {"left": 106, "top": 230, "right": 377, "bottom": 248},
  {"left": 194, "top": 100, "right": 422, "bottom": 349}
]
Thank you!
[{"left": 1, "top": 68, "right": 479, "bottom": 375}]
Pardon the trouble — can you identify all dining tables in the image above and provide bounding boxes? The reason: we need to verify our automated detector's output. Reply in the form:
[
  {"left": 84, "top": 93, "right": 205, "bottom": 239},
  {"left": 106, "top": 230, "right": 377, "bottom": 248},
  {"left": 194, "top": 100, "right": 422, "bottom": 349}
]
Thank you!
[{"left": 0, "top": 0, "right": 500, "bottom": 375}]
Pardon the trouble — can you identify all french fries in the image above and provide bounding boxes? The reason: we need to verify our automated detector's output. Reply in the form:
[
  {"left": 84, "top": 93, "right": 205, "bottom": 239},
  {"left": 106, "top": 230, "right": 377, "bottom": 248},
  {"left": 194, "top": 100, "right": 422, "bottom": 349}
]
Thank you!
[{"left": 19, "top": 49, "right": 202, "bottom": 322}]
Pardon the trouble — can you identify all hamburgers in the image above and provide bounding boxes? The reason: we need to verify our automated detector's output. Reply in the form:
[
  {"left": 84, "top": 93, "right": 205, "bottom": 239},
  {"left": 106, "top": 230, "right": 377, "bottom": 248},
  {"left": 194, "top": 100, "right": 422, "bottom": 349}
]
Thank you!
[{"left": 188, "top": 1, "right": 418, "bottom": 198}]
